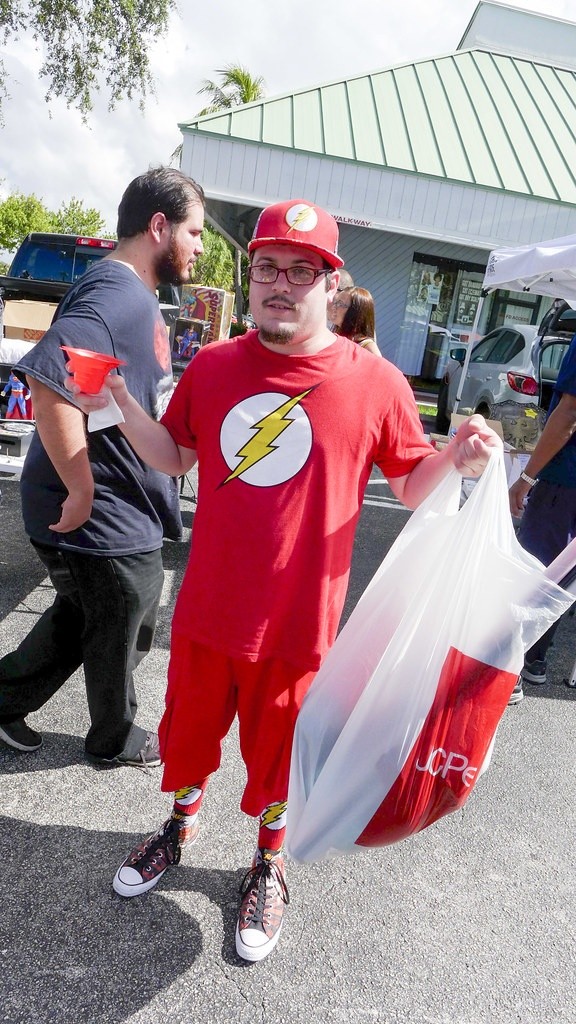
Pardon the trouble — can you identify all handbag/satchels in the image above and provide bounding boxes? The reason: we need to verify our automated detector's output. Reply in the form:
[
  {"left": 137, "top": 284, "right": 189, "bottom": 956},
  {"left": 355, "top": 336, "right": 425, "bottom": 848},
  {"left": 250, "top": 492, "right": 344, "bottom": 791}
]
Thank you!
[{"left": 283, "top": 437, "right": 576, "bottom": 865}]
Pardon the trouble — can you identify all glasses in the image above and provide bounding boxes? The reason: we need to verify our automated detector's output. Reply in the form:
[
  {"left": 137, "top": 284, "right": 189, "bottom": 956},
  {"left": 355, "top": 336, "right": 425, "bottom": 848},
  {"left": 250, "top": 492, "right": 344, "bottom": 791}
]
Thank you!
[
  {"left": 334, "top": 300, "right": 351, "bottom": 309},
  {"left": 247, "top": 262, "right": 332, "bottom": 286}
]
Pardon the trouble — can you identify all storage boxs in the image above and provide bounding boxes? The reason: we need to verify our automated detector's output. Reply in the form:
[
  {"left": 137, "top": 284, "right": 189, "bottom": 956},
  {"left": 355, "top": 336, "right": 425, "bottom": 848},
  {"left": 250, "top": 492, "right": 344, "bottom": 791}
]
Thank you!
[
  {"left": 172, "top": 317, "right": 211, "bottom": 361},
  {"left": 2, "top": 299, "right": 59, "bottom": 346},
  {"left": 180, "top": 283, "right": 236, "bottom": 342}
]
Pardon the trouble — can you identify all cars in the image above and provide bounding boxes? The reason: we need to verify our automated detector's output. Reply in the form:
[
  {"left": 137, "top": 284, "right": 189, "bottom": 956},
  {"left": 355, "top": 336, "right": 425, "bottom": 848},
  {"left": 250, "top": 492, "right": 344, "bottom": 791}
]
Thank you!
[{"left": 435, "top": 322, "right": 576, "bottom": 435}]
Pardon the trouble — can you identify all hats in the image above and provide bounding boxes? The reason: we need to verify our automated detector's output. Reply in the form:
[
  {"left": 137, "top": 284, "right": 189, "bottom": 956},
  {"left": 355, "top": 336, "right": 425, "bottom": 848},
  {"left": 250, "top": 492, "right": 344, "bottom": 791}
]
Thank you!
[{"left": 247, "top": 198, "right": 344, "bottom": 268}]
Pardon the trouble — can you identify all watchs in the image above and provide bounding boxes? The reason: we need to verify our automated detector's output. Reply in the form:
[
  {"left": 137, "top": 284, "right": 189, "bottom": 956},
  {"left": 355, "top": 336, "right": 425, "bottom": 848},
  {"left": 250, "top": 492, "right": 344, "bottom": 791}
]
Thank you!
[{"left": 519, "top": 471, "right": 539, "bottom": 487}]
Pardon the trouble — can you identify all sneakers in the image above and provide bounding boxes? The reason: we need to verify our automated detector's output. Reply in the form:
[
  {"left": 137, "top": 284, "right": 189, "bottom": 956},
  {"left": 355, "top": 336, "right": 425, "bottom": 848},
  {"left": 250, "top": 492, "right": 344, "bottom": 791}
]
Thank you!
[
  {"left": 113, "top": 813, "right": 200, "bottom": 897},
  {"left": 507, "top": 676, "right": 523, "bottom": 705},
  {"left": 0, "top": 719, "right": 43, "bottom": 754},
  {"left": 235, "top": 850, "right": 290, "bottom": 962},
  {"left": 120, "top": 730, "right": 161, "bottom": 777},
  {"left": 521, "top": 655, "right": 547, "bottom": 685}
]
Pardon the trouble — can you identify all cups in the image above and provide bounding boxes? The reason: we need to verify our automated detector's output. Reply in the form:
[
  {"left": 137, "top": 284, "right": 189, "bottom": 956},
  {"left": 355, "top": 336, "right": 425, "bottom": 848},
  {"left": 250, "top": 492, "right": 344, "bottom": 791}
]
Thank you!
[{"left": 58, "top": 346, "right": 127, "bottom": 393}]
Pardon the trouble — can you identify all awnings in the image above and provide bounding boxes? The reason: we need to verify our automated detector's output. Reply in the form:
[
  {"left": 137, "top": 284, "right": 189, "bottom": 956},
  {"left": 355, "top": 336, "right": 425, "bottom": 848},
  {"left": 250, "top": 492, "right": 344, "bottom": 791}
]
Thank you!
[{"left": 445, "top": 233, "right": 576, "bottom": 465}]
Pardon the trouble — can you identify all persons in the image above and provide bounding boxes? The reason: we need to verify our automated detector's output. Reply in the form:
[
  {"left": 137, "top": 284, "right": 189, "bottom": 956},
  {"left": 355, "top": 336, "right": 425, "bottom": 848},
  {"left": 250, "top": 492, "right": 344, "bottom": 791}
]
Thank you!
[
  {"left": 507, "top": 321, "right": 575, "bottom": 708},
  {"left": 63, "top": 198, "right": 505, "bottom": 966},
  {"left": 1, "top": 374, "right": 31, "bottom": 420},
  {"left": 0, "top": 168, "right": 205, "bottom": 766},
  {"left": 326, "top": 269, "right": 382, "bottom": 357}
]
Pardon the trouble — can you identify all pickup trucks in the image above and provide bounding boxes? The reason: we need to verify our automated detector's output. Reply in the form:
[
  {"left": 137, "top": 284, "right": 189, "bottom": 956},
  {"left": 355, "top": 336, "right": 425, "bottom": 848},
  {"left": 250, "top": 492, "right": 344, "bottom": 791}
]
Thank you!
[{"left": 1, "top": 229, "right": 182, "bottom": 397}]
